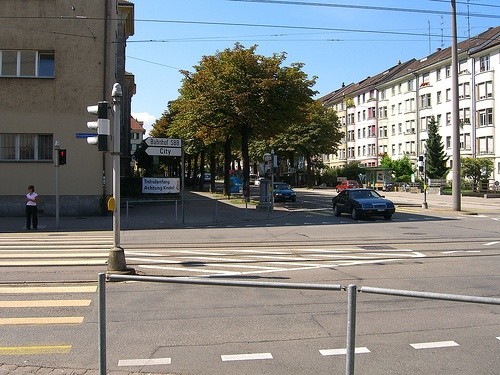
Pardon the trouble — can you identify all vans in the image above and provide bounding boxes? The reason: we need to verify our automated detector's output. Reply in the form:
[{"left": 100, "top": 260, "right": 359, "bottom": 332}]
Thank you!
[{"left": 249, "top": 174, "right": 258, "bottom": 184}]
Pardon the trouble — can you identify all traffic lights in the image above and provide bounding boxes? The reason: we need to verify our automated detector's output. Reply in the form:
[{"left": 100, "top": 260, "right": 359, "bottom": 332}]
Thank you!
[
  {"left": 87, "top": 100, "right": 108, "bottom": 153},
  {"left": 58, "top": 148, "right": 67, "bottom": 167}
]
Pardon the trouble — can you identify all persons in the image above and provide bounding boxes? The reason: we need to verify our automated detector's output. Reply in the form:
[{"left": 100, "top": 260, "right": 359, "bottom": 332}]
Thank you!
[{"left": 25, "top": 185, "right": 39, "bottom": 231}]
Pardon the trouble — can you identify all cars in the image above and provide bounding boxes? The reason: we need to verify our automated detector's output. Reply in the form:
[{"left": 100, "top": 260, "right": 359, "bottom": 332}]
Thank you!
[
  {"left": 331, "top": 189, "right": 395, "bottom": 221},
  {"left": 335, "top": 179, "right": 359, "bottom": 193},
  {"left": 199, "top": 173, "right": 213, "bottom": 182},
  {"left": 272, "top": 182, "right": 296, "bottom": 203}
]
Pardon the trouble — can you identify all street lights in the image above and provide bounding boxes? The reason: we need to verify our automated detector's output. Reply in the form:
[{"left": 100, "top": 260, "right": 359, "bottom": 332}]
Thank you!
[
  {"left": 406, "top": 68, "right": 419, "bottom": 179},
  {"left": 343, "top": 98, "right": 350, "bottom": 164},
  {"left": 373, "top": 86, "right": 380, "bottom": 168}
]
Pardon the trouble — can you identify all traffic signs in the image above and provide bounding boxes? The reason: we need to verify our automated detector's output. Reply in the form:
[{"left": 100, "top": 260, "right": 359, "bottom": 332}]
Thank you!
[
  {"left": 145, "top": 146, "right": 182, "bottom": 157},
  {"left": 145, "top": 138, "right": 182, "bottom": 147},
  {"left": 143, "top": 177, "right": 181, "bottom": 194}
]
